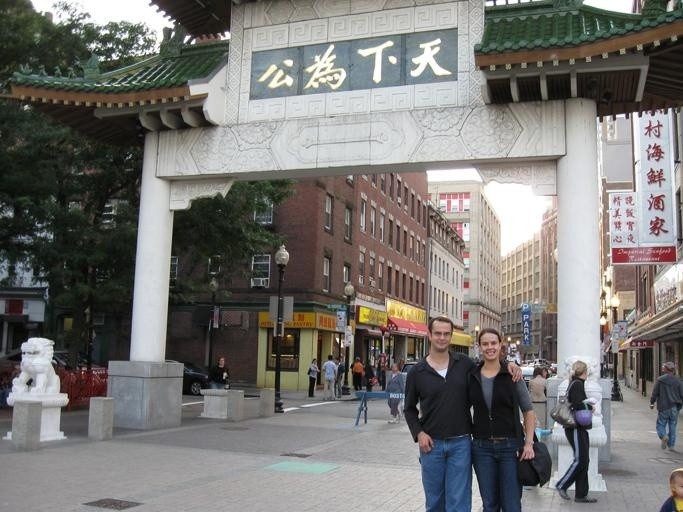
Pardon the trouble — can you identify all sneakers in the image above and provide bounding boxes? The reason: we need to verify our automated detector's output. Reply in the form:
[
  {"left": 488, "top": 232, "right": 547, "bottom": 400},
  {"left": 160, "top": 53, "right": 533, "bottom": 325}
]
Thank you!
[
  {"left": 387, "top": 418, "right": 400, "bottom": 424},
  {"left": 573, "top": 495, "right": 598, "bottom": 503},
  {"left": 661, "top": 434, "right": 670, "bottom": 450},
  {"left": 555, "top": 484, "right": 571, "bottom": 500}
]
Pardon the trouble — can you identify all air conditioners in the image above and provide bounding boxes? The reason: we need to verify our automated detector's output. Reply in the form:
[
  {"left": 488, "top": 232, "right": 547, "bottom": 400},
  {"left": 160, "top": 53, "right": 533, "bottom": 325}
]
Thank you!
[
  {"left": 251, "top": 278, "right": 265, "bottom": 287},
  {"left": 370, "top": 281, "right": 374, "bottom": 287},
  {"left": 358, "top": 275, "right": 363, "bottom": 284}
]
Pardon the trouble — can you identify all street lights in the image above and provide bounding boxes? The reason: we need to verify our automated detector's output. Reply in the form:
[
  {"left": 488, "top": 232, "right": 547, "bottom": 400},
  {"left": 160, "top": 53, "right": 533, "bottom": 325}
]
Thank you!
[
  {"left": 600, "top": 294, "right": 625, "bottom": 405},
  {"left": 507, "top": 336, "right": 511, "bottom": 363},
  {"left": 516, "top": 339, "right": 520, "bottom": 351},
  {"left": 474, "top": 325, "right": 479, "bottom": 357},
  {"left": 270, "top": 242, "right": 289, "bottom": 413},
  {"left": 341, "top": 278, "right": 353, "bottom": 397},
  {"left": 208, "top": 275, "right": 219, "bottom": 390}
]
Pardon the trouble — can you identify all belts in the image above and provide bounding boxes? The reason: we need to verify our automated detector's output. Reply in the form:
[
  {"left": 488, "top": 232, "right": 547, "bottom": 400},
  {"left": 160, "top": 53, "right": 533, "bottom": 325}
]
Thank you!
[{"left": 478, "top": 434, "right": 516, "bottom": 440}]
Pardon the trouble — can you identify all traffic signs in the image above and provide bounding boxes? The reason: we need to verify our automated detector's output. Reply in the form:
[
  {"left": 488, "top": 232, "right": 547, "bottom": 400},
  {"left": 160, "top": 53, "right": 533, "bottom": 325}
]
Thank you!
[{"left": 324, "top": 303, "right": 345, "bottom": 310}]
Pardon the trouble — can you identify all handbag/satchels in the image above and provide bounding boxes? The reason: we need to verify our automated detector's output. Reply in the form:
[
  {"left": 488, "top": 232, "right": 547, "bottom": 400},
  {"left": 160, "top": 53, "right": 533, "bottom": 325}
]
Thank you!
[
  {"left": 550, "top": 397, "right": 576, "bottom": 429},
  {"left": 574, "top": 408, "right": 593, "bottom": 426},
  {"left": 514, "top": 439, "right": 553, "bottom": 488}
]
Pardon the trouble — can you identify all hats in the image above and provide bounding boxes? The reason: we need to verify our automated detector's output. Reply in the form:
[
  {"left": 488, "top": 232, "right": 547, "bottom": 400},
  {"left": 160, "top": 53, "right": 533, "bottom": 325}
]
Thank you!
[{"left": 662, "top": 361, "right": 675, "bottom": 369}]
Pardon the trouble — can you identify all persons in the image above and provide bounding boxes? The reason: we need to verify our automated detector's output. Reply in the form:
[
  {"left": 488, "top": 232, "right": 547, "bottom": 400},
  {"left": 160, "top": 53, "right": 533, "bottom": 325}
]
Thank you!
[
  {"left": 206, "top": 356, "right": 231, "bottom": 390},
  {"left": 306, "top": 353, "right": 405, "bottom": 426},
  {"left": 550, "top": 367, "right": 556, "bottom": 377},
  {"left": 657, "top": 467, "right": 683, "bottom": 511},
  {"left": 648, "top": 361, "right": 683, "bottom": 451},
  {"left": 541, "top": 367, "right": 548, "bottom": 379},
  {"left": 465, "top": 326, "right": 538, "bottom": 512},
  {"left": 527, "top": 367, "right": 547, "bottom": 428},
  {"left": 554, "top": 359, "right": 598, "bottom": 504},
  {"left": 401, "top": 315, "right": 525, "bottom": 512}
]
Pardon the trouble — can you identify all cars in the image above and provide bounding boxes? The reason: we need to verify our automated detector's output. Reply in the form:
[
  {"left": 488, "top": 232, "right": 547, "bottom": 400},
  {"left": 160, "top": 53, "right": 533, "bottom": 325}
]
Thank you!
[
  {"left": 165, "top": 360, "right": 230, "bottom": 395},
  {"left": 519, "top": 358, "right": 557, "bottom": 388},
  {"left": 399, "top": 362, "right": 418, "bottom": 374},
  {"left": 0, "top": 347, "right": 107, "bottom": 388}
]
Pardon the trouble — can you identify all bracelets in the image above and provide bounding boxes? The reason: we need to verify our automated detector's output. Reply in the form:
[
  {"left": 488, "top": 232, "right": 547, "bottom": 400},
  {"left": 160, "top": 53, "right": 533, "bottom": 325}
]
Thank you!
[{"left": 523, "top": 438, "right": 535, "bottom": 445}]
